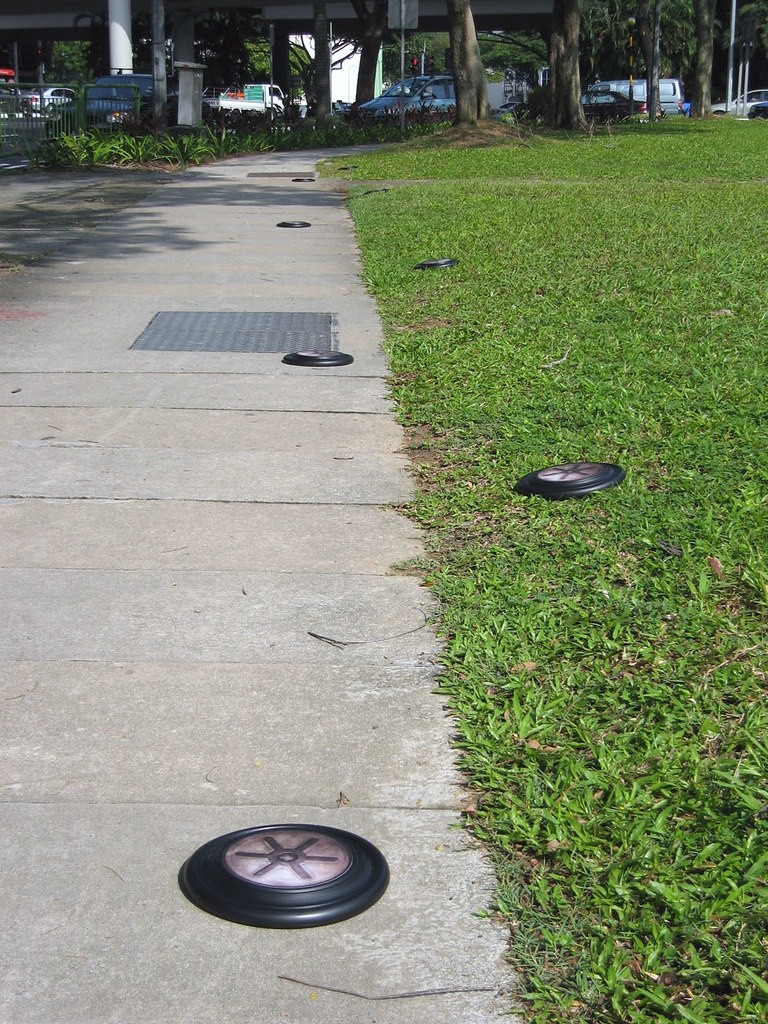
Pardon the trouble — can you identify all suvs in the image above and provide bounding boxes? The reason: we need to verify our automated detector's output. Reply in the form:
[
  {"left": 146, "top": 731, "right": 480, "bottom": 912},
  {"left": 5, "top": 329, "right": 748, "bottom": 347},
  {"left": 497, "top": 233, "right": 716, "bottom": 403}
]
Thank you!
[
  {"left": 357, "top": 74, "right": 456, "bottom": 118},
  {"left": 45, "top": 68, "right": 212, "bottom": 148}
]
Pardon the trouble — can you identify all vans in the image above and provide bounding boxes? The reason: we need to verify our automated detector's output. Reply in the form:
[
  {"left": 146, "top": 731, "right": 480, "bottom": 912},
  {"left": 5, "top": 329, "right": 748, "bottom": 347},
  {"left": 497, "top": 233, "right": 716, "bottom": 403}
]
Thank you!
[{"left": 581, "top": 79, "right": 684, "bottom": 115}]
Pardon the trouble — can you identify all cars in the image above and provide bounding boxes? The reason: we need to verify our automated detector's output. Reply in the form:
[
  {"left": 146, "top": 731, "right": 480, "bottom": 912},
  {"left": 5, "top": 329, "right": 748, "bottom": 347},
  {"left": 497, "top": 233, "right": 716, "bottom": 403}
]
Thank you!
[
  {"left": 19, "top": 88, "right": 75, "bottom": 114},
  {"left": 711, "top": 89, "right": 768, "bottom": 116},
  {"left": 495, "top": 102, "right": 519, "bottom": 115},
  {"left": 748, "top": 102, "right": 768, "bottom": 119},
  {"left": 0, "top": 88, "right": 22, "bottom": 110},
  {"left": 581, "top": 90, "right": 648, "bottom": 126}
]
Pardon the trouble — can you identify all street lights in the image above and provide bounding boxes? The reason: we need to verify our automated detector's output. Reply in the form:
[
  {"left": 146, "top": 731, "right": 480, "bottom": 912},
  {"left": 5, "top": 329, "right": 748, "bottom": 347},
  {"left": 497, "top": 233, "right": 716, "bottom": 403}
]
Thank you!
[{"left": 618, "top": 18, "right": 636, "bottom": 116}]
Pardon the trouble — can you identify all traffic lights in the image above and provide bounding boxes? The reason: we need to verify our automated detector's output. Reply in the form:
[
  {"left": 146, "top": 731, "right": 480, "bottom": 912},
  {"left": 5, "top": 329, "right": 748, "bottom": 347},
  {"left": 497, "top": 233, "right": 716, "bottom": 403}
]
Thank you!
[
  {"left": 424, "top": 53, "right": 434, "bottom": 76},
  {"left": 413, "top": 57, "right": 418, "bottom": 72}
]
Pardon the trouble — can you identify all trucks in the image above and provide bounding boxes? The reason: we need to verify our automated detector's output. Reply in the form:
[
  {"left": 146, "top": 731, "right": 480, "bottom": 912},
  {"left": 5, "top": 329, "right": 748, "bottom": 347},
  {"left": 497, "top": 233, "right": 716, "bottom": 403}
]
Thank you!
[{"left": 201, "top": 84, "right": 284, "bottom": 122}]
